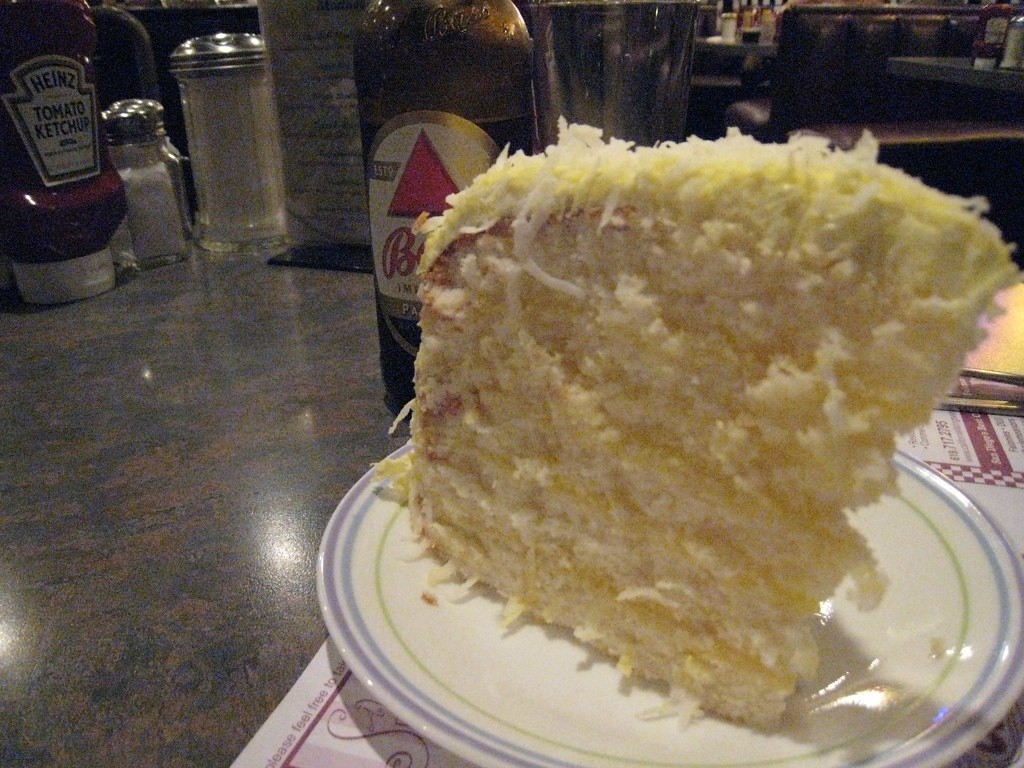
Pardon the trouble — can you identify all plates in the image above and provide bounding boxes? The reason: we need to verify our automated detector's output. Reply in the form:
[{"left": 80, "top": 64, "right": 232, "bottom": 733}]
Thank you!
[{"left": 313, "top": 437, "right": 1024, "bottom": 768}]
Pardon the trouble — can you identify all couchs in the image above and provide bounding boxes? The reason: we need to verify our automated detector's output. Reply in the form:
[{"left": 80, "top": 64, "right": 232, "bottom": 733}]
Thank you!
[{"left": 723, "top": 0, "right": 1024, "bottom": 189}]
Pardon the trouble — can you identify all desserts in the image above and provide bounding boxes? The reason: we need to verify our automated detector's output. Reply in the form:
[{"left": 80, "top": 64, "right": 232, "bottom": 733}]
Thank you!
[{"left": 370, "top": 114, "right": 1023, "bottom": 737}]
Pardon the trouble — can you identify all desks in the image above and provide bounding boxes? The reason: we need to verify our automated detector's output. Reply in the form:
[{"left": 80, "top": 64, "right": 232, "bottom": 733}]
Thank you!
[{"left": 0, "top": 232, "right": 1024, "bottom": 768}]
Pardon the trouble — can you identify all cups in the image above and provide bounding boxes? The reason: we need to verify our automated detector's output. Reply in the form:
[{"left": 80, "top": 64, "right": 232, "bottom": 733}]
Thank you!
[
  {"left": 529, "top": 0, "right": 701, "bottom": 151},
  {"left": 741, "top": 31, "right": 761, "bottom": 43}
]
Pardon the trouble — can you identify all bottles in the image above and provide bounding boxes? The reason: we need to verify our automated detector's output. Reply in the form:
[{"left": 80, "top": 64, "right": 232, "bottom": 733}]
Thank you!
[
  {"left": 0, "top": 0, "right": 284, "bottom": 306},
  {"left": 973, "top": 4, "right": 1011, "bottom": 71},
  {"left": 348, "top": 0, "right": 534, "bottom": 419}
]
